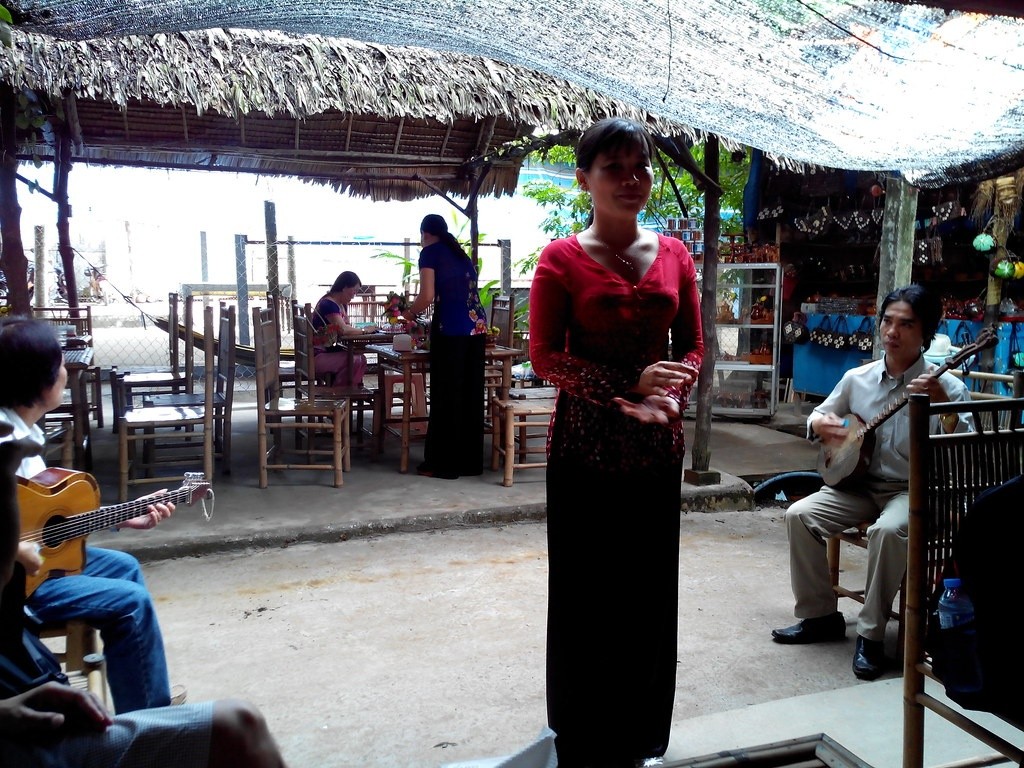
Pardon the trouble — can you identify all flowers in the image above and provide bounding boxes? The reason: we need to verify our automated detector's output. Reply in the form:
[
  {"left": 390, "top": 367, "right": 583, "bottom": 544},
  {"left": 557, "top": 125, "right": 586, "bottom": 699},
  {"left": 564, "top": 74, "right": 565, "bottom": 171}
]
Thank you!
[{"left": 380, "top": 292, "right": 423, "bottom": 325}]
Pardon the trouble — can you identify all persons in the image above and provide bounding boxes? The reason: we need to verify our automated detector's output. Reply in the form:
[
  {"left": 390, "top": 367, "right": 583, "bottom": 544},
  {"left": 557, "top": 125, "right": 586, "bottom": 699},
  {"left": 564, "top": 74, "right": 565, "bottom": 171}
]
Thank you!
[
  {"left": 772, "top": 285, "right": 973, "bottom": 682},
  {"left": 0, "top": 468, "right": 287, "bottom": 768},
  {"left": 531, "top": 118, "right": 705, "bottom": 768},
  {"left": 0, "top": 315, "right": 187, "bottom": 715},
  {"left": 311, "top": 272, "right": 366, "bottom": 388},
  {"left": 403, "top": 214, "right": 487, "bottom": 480}
]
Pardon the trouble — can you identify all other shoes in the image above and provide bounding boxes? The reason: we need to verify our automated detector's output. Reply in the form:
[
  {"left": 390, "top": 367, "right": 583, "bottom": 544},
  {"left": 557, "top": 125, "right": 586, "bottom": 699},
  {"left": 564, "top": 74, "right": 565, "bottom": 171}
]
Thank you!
[{"left": 416, "top": 461, "right": 459, "bottom": 479}]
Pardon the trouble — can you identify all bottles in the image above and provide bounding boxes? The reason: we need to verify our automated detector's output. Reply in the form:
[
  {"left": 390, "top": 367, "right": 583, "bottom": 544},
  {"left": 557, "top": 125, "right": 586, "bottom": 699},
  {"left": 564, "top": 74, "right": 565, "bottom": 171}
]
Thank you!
[{"left": 938, "top": 578, "right": 976, "bottom": 630}]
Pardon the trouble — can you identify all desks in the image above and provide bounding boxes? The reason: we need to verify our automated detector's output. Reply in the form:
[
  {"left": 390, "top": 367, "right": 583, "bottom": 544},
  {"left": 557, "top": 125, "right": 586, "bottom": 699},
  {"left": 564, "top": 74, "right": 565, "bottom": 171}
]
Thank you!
[
  {"left": 365, "top": 343, "right": 524, "bottom": 472},
  {"left": 35, "top": 347, "right": 95, "bottom": 472},
  {"left": 792, "top": 311, "right": 1024, "bottom": 423},
  {"left": 340, "top": 329, "right": 407, "bottom": 443}
]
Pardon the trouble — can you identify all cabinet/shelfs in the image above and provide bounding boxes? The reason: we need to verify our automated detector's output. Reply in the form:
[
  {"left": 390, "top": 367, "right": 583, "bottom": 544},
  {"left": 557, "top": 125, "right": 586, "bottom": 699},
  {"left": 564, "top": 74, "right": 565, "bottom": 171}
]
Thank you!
[{"left": 683, "top": 262, "right": 785, "bottom": 422}]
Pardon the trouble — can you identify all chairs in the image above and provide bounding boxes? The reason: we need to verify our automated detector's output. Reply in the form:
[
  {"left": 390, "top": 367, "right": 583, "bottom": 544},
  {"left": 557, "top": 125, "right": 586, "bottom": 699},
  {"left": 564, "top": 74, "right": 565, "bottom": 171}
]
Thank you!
[
  {"left": 492, "top": 396, "right": 557, "bottom": 486},
  {"left": 829, "top": 367, "right": 1024, "bottom": 768},
  {"left": 27, "top": 623, "right": 97, "bottom": 691},
  {"left": 509, "top": 387, "right": 556, "bottom": 464},
  {"left": 292, "top": 300, "right": 379, "bottom": 464},
  {"left": 143, "top": 302, "right": 235, "bottom": 475},
  {"left": 484, "top": 293, "right": 515, "bottom": 434},
  {"left": 118, "top": 294, "right": 193, "bottom": 442},
  {"left": 30, "top": 305, "right": 103, "bottom": 428},
  {"left": 109, "top": 292, "right": 178, "bottom": 433},
  {"left": 121, "top": 306, "right": 213, "bottom": 505},
  {"left": 266, "top": 292, "right": 326, "bottom": 446},
  {"left": 253, "top": 304, "right": 351, "bottom": 487}
]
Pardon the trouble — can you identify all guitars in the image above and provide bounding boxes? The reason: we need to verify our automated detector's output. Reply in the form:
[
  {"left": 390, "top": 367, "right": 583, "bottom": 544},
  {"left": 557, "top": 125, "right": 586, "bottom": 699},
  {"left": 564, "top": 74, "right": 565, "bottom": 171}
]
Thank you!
[
  {"left": 816, "top": 325, "right": 1000, "bottom": 493},
  {"left": 14, "top": 467, "right": 215, "bottom": 602}
]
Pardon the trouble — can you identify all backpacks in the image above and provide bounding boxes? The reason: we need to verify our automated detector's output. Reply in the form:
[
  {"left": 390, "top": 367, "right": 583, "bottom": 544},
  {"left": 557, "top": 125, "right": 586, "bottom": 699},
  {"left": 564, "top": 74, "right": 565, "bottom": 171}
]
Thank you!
[{"left": 926, "top": 472, "right": 1024, "bottom": 728}]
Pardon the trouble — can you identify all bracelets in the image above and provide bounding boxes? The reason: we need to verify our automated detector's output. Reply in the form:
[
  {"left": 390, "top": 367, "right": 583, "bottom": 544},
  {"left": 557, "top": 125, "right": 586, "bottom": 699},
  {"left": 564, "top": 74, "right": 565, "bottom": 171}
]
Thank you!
[
  {"left": 940, "top": 410, "right": 956, "bottom": 423},
  {"left": 111, "top": 527, "right": 119, "bottom": 532},
  {"left": 408, "top": 308, "right": 415, "bottom": 317}
]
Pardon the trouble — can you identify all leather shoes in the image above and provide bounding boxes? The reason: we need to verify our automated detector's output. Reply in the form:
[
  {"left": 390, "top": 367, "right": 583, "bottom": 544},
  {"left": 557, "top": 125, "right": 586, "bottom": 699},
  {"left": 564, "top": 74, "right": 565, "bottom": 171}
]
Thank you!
[
  {"left": 851, "top": 634, "right": 887, "bottom": 679},
  {"left": 772, "top": 611, "right": 848, "bottom": 644}
]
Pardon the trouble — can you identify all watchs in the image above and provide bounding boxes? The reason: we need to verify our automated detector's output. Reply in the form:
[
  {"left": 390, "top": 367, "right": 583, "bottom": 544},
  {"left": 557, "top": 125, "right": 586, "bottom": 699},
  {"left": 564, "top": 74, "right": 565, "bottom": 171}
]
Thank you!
[{"left": 361, "top": 328, "right": 365, "bottom": 335}]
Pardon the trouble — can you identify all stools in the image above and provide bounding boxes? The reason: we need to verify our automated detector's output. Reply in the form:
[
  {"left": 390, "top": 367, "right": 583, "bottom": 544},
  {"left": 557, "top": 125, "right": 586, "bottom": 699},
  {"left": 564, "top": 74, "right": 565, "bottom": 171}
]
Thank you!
[{"left": 385, "top": 371, "right": 428, "bottom": 434}]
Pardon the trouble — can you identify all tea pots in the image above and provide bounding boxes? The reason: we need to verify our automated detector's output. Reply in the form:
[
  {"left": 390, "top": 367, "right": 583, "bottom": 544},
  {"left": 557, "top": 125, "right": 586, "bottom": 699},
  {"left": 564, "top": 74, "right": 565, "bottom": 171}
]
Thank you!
[{"left": 717, "top": 301, "right": 734, "bottom": 324}]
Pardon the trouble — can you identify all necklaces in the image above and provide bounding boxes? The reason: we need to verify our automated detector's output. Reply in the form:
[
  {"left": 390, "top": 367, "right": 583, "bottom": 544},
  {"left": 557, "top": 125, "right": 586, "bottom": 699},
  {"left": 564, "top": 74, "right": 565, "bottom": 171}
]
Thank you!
[{"left": 587, "top": 227, "right": 641, "bottom": 271}]
